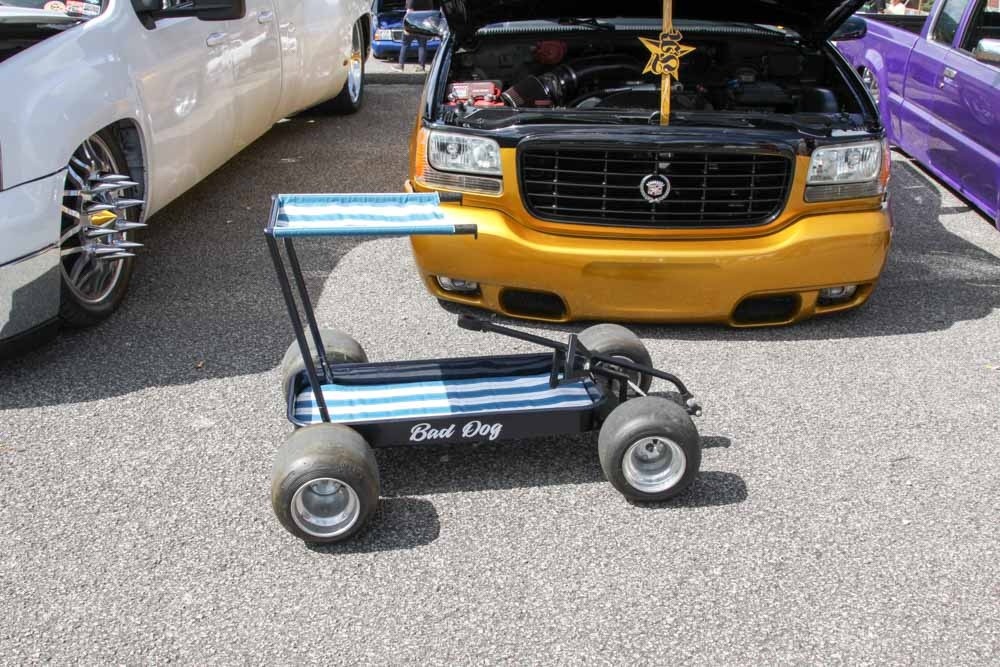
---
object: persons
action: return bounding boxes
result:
[392,0,435,73]
[860,0,925,15]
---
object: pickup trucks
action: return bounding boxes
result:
[829,0,1000,230]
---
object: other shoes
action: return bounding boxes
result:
[416,66,426,73]
[391,63,404,72]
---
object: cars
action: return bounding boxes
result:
[370,0,451,61]
[404,0,897,326]
[0,1,378,353]
